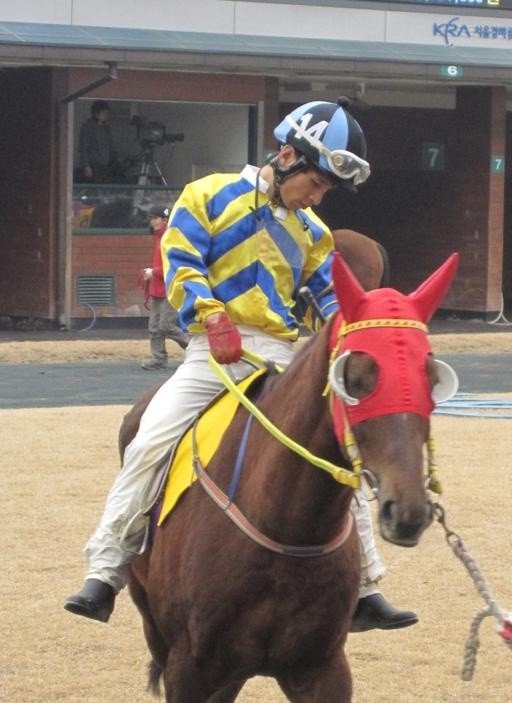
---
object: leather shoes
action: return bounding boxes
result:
[349,594,418,632]
[64,578,115,623]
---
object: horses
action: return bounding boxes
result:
[331,227,391,292]
[118,251,461,703]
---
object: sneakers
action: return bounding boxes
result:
[142,359,167,369]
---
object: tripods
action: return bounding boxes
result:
[131,146,176,216]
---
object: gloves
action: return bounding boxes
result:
[203,312,242,365]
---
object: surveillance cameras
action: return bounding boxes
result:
[353,80,366,100]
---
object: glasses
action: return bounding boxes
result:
[326,150,371,185]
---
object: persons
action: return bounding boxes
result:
[63,95,419,633]
[136,205,190,371]
[80,98,122,183]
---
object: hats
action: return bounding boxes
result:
[149,206,170,218]
[274,96,368,193]
